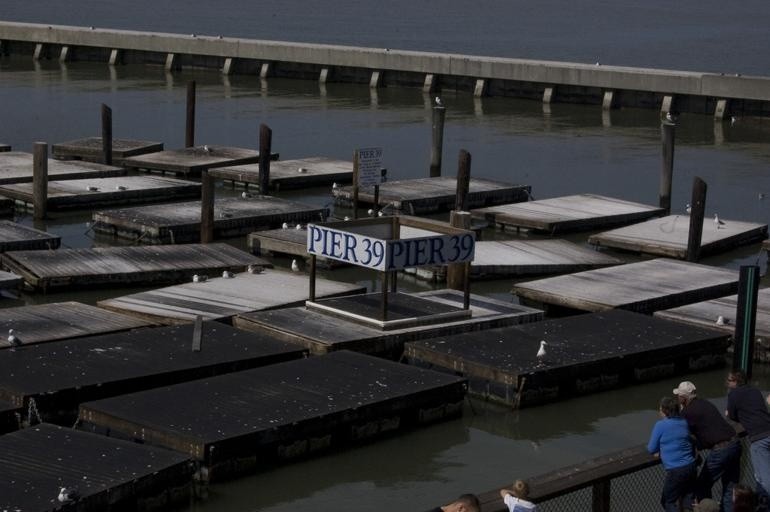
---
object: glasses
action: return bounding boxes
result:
[727,378,736,381]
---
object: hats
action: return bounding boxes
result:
[672,380,697,399]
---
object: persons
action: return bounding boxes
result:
[730,483,753,512]
[724,367,770,511]
[648,395,696,511]
[500,479,538,511]
[428,493,482,511]
[671,379,742,511]
[690,496,720,511]
